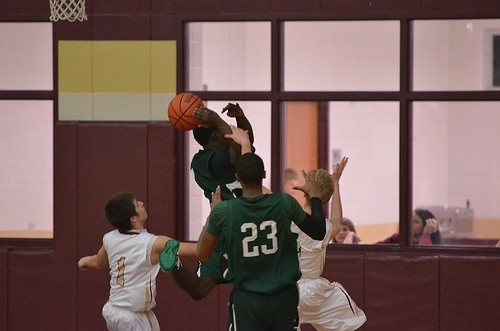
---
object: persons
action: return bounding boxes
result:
[377,209,442,245]
[159,102,367,331]
[78,191,198,331]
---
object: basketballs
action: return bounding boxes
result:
[167,92,204,132]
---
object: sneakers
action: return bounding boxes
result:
[159,239,183,272]
